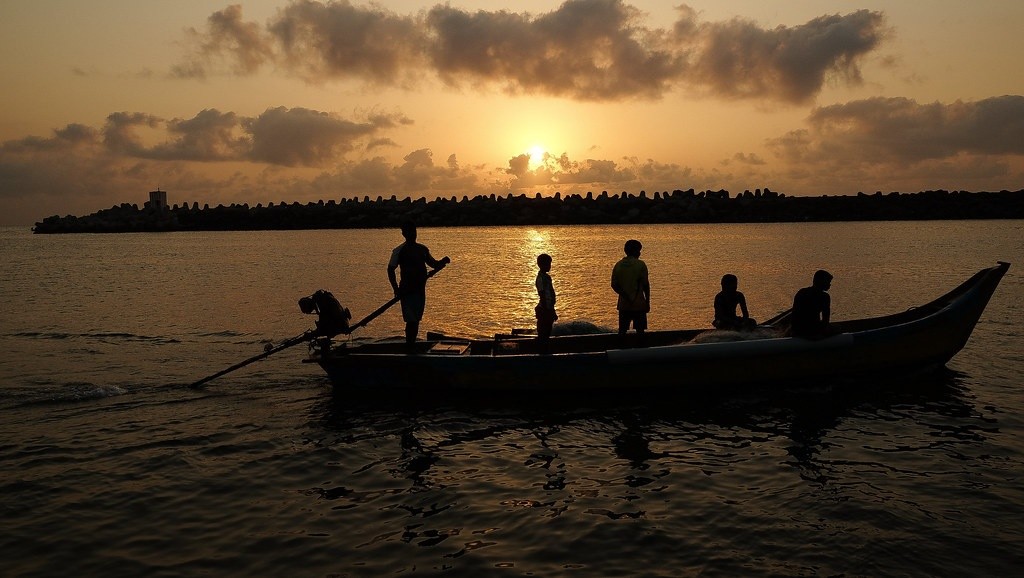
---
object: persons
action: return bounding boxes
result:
[387,224,446,354]
[535,254,558,353]
[714,274,757,332]
[611,240,650,349]
[791,269,840,340]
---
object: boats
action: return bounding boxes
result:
[299,259,1012,393]
